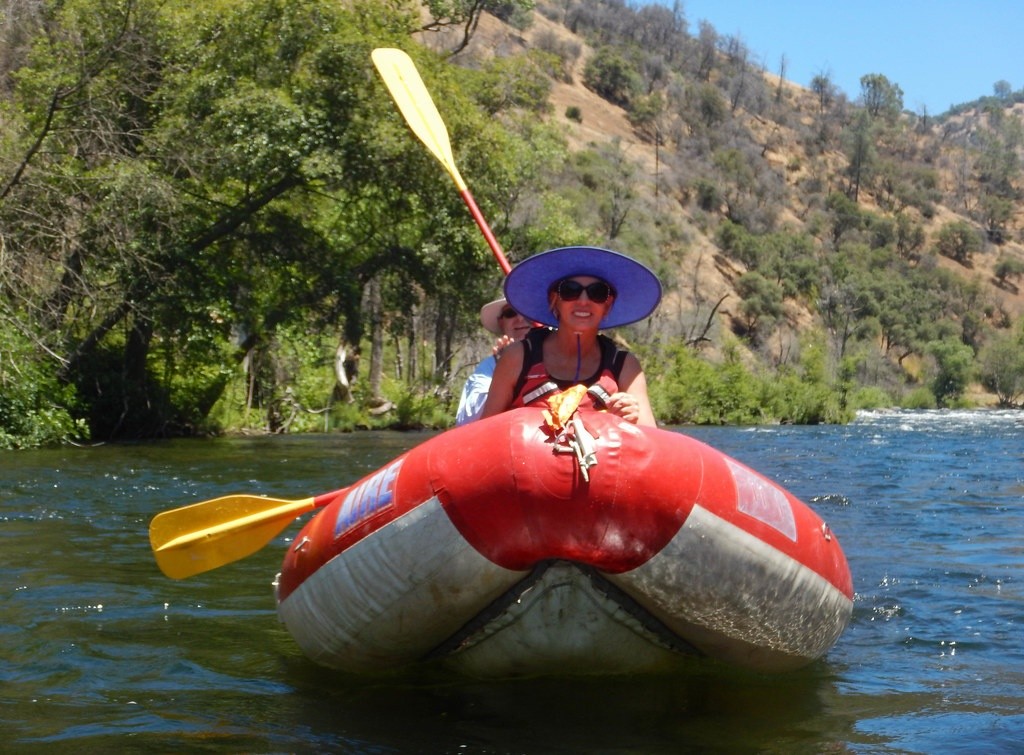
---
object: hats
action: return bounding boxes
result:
[480,298,509,338]
[504,246,662,330]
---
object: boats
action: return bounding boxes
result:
[271,407,852,682]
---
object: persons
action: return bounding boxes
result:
[455,298,560,425]
[481,246,662,430]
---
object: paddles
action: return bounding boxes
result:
[148,488,345,581]
[370,45,514,276]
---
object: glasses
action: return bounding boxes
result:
[548,279,617,305]
[498,308,518,319]
